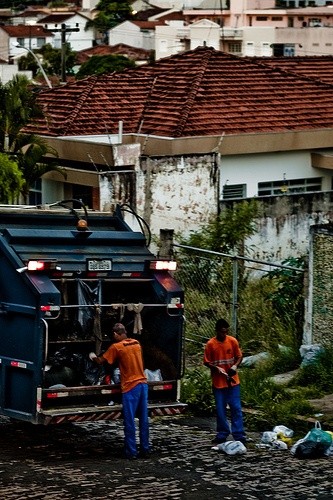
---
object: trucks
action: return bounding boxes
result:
[1,201,190,428]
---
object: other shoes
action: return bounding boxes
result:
[211,437,227,444]
[235,437,255,444]
[119,450,138,460]
[139,449,151,459]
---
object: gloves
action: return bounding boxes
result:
[216,364,238,378]
[88,351,97,361]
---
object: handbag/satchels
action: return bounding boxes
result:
[211,440,247,456]
[255,421,333,460]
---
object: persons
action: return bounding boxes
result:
[203,317,248,446]
[89,322,150,461]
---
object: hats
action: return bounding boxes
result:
[215,319,230,327]
[113,322,128,334]
